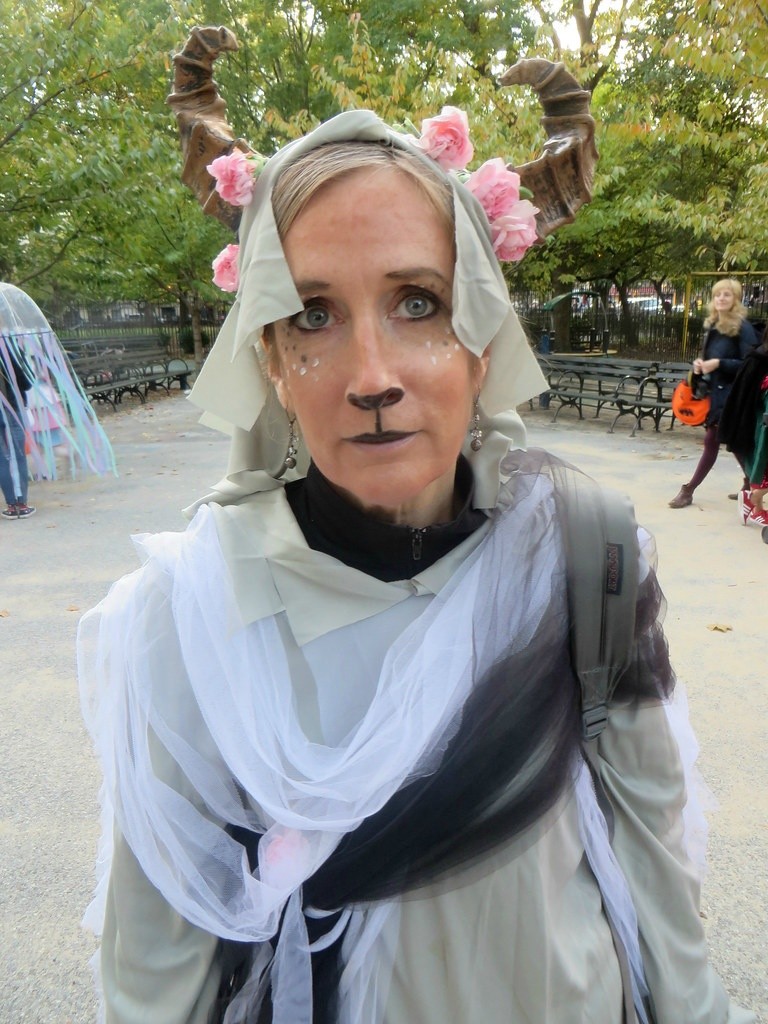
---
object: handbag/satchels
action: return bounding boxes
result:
[672,365,710,426]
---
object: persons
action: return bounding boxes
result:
[669,278,767,525]
[98,108,724,1024]
[0,280,49,521]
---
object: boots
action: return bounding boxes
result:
[728,478,750,500]
[668,484,692,507]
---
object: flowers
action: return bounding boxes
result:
[212,244,241,292]
[206,149,270,207]
[490,199,541,262]
[457,156,535,224]
[420,105,474,172]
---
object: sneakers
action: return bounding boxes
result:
[750,510,768,526]
[18,506,36,517]
[2,508,18,519]
[738,490,756,525]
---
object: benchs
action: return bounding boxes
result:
[61,333,195,413]
[536,355,655,434]
[538,359,679,431]
[630,362,694,437]
